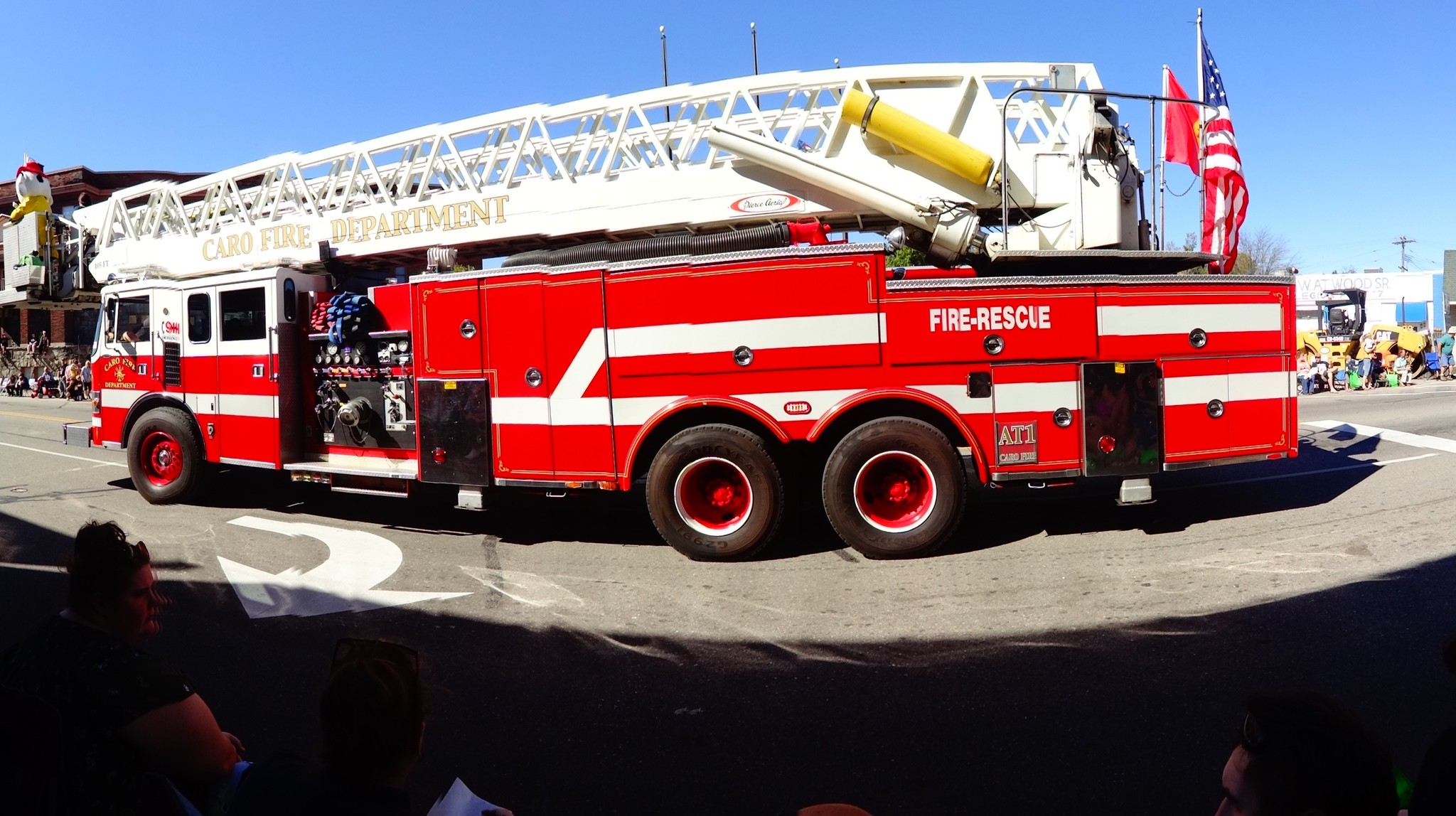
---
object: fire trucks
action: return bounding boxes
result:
[10,10,1301,560]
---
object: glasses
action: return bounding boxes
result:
[1315,356,1321,359]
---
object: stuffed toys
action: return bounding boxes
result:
[10,161,59,262]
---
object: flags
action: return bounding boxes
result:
[1200,28,1249,276]
[1163,69,1200,177]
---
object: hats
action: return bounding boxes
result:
[1448,326,1456,333]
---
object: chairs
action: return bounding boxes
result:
[1300,370,1346,392]
[0,378,35,397]
[1425,352,1441,380]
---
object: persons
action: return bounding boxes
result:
[120,318,150,342]
[1297,326,1456,395]
[0,330,92,401]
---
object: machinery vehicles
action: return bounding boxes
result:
[1296,286,1432,379]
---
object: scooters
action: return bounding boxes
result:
[30,376,60,399]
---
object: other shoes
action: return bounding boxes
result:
[1363,386,1368,391]
[1303,392,1308,395]
[1368,385,1374,390]
[1308,392,1312,394]
[44,353,46,356]
[1330,389,1338,393]
[1406,383,1411,387]
[1399,382,1405,387]
[1440,376,1447,381]
[33,353,34,355]
[27,353,30,355]
[1323,371,1327,377]
[1446,375,1453,380]
[0,393,23,397]
[3,353,5,356]
[66,394,91,401]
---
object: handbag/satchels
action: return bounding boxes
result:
[1386,366,1398,387]
[1348,367,1362,388]
